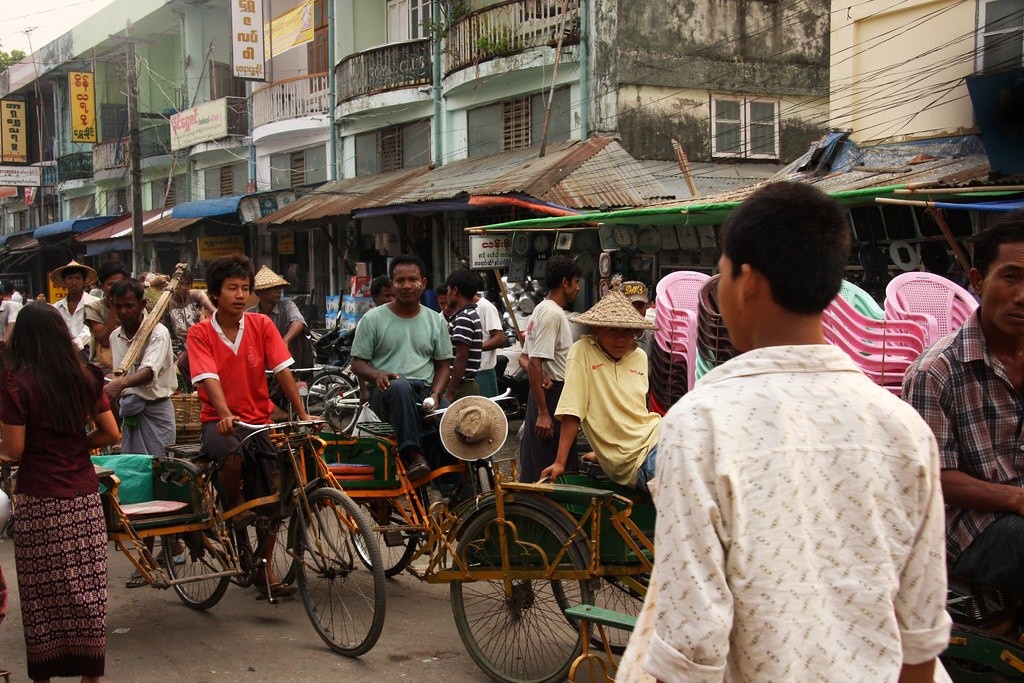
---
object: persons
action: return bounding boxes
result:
[246,265,316,405]
[901,223,1024,618]
[623,279,657,378]
[614,181,955,683]
[50,259,216,588]
[518,254,580,484]
[0,279,47,342]
[0,302,120,683]
[350,253,505,497]
[186,253,325,592]
[540,290,662,490]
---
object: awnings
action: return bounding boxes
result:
[0,132,1024,256]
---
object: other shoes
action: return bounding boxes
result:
[408,459,431,479]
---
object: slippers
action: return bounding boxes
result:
[254,582,297,600]
[233,510,256,533]
[155,556,186,568]
[125,571,149,588]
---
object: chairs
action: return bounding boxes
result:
[540,466,659,564]
[87,452,192,518]
[643,272,982,417]
[312,430,403,491]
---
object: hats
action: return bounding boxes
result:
[438,395,509,463]
[253,264,291,290]
[49,259,99,288]
[569,275,659,330]
[619,282,651,304]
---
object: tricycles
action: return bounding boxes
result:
[97,314,1024,683]
[91,417,387,658]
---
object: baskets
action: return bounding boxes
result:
[171,394,203,430]
